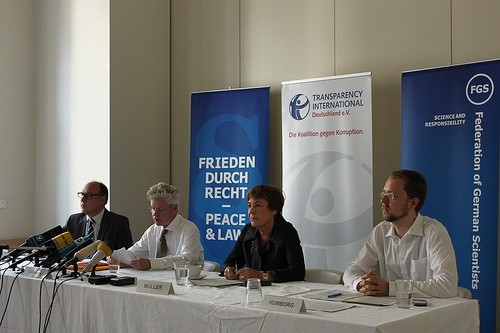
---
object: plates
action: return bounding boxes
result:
[188,275,207,280]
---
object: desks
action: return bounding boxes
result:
[0,254,478,333]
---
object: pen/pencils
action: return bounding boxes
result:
[328,293,342,298]
[235,260,238,272]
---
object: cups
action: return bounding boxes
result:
[108,255,120,276]
[395,280,414,310]
[246,277,263,308]
[189,265,202,277]
[173,261,190,287]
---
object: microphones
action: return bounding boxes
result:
[2,225,114,276]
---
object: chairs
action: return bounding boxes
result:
[203,261,221,272]
[303,269,343,284]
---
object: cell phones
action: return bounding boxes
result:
[413,300,427,307]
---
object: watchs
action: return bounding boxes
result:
[262,270,268,282]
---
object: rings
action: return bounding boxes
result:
[239,269,243,271]
[241,276,244,279]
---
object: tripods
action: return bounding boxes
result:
[64,262,90,281]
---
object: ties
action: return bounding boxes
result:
[87,219,95,240]
[159,229,169,257]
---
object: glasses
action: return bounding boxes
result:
[380,191,409,200]
[76,192,103,200]
[150,207,170,212]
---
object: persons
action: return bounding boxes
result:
[342,169,459,298]
[107,182,204,270]
[63,181,133,261]
[218,185,306,283]
